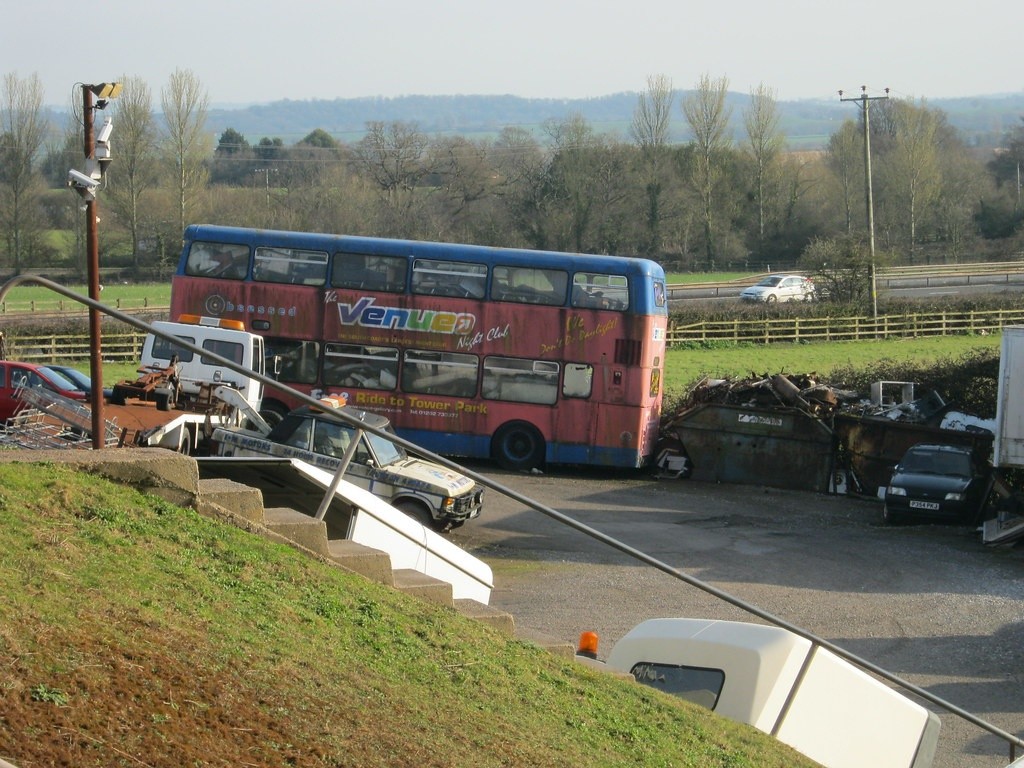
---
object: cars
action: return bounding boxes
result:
[0,361,115,425]
[885,446,979,523]
[739,275,816,304]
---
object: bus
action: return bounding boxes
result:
[170,222,667,472]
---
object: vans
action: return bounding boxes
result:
[137,313,265,413]
[575,617,942,768]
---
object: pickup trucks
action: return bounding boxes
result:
[211,384,485,532]
